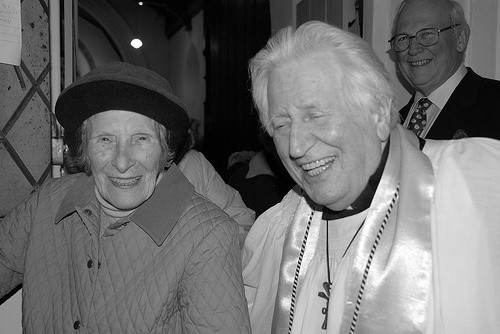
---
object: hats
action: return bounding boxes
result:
[55,61,192,164]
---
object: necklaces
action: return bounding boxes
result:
[318,210,366,330]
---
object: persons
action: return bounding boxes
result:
[0,62,254,334]
[226,0,500,334]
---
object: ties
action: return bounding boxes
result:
[406,97,433,137]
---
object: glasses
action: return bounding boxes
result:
[388,23,462,53]
